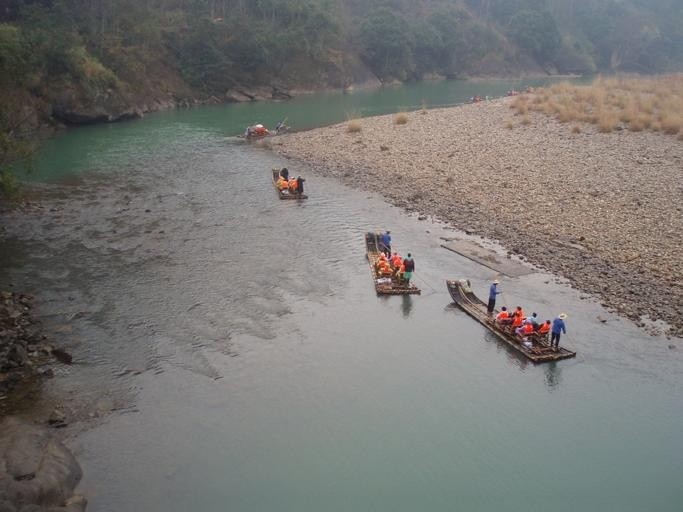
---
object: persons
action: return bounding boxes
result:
[276,167,305,195]
[496,306,567,348]
[377,231,415,290]
[487,280,500,313]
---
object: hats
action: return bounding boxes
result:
[558,313,568,320]
[492,279,500,284]
[385,228,392,232]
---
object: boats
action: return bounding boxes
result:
[224,113,289,143]
[272,167,309,201]
[446,278,577,364]
[363,229,421,296]
[465,82,532,104]
